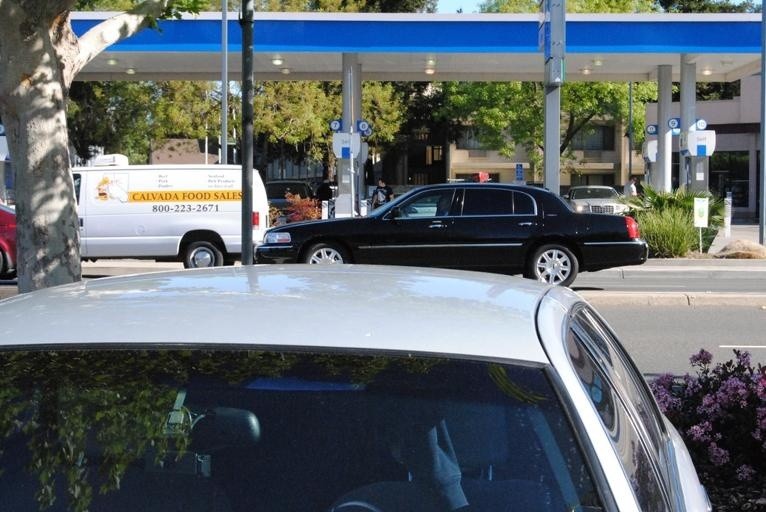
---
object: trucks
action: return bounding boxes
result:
[67,166,271,267]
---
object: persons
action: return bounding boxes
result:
[371,177,395,210]
[624,176,640,197]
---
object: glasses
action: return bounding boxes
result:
[633,179,637,182]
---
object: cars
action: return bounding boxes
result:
[0,263,714,512]
[254,182,648,286]
[563,186,631,216]
[265,178,336,224]
